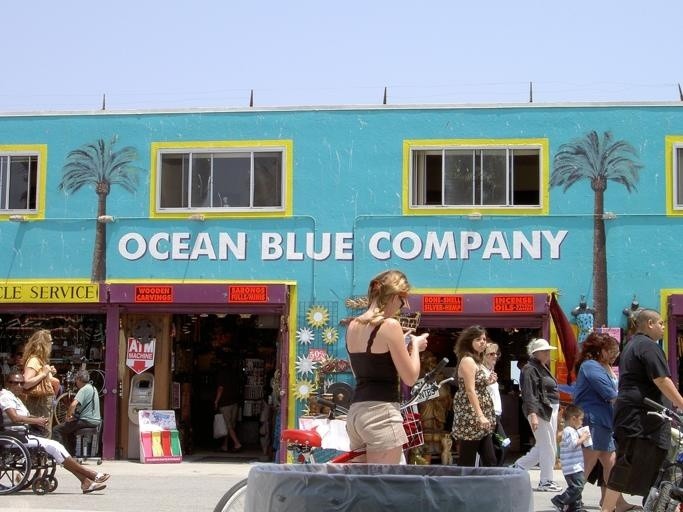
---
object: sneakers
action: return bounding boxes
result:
[538,479,563,492]
[551,499,568,511]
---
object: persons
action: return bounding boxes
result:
[571,299,597,344]
[344,268,431,466]
[588,335,621,507]
[479,341,508,467]
[550,403,592,512]
[212,355,244,454]
[599,307,683,512]
[449,321,499,467]
[567,331,644,512]
[506,337,564,492]
[622,300,646,343]
[0,325,112,494]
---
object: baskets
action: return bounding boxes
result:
[399,407,425,451]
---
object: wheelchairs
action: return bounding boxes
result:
[0,407,58,494]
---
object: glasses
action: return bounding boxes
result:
[6,380,25,386]
[399,296,406,308]
[485,352,501,357]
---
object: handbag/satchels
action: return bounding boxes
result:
[64,413,80,428]
[213,407,228,439]
[21,355,55,400]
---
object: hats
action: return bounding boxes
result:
[532,339,557,354]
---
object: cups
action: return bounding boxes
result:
[577,426,593,448]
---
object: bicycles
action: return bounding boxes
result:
[56,372,77,425]
[209,356,453,512]
[637,399,681,512]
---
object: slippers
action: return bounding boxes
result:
[95,472,111,483]
[82,481,107,494]
